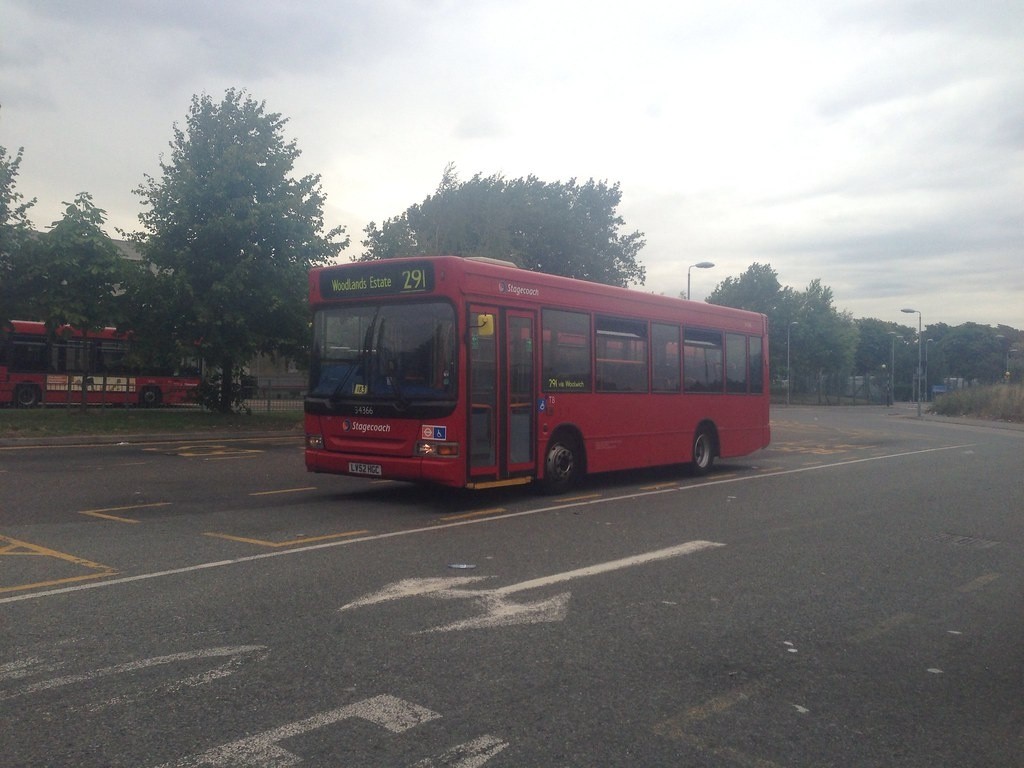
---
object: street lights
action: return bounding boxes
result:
[901,308,923,417]
[786,322,800,405]
[686,262,716,301]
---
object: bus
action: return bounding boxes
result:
[305,256,770,497]
[0,318,203,408]
[389,321,725,411]
